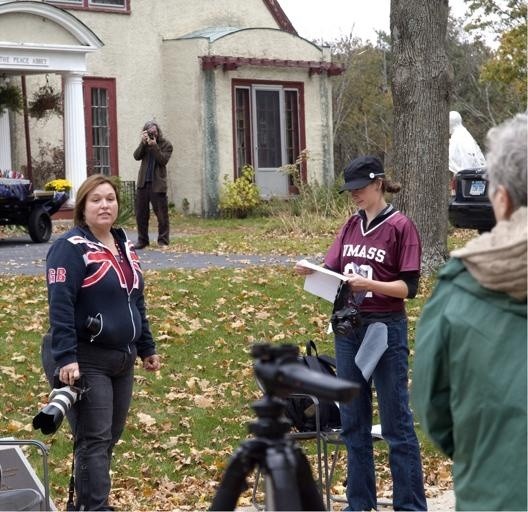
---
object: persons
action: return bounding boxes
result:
[131,120,173,249]
[446,112,486,197]
[411,108,527,511]
[294,156,432,512]
[41,171,162,511]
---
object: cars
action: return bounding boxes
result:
[448,167,497,234]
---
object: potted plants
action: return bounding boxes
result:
[1,81,63,121]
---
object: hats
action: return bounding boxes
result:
[338,157,386,194]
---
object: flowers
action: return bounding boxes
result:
[44,178,72,193]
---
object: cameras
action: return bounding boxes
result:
[330,306,361,335]
[148,131,157,140]
[32,367,88,435]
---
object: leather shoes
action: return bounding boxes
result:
[135,242,147,249]
[158,241,168,248]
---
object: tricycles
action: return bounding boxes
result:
[0,178,71,245]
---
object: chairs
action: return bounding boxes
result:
[250,355,394,512]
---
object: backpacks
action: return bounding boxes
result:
[289,339,341,432]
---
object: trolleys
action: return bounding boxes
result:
[0,436,59,511]
